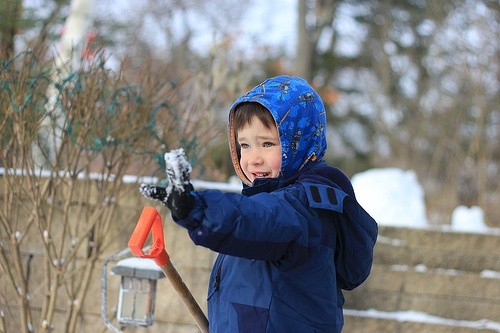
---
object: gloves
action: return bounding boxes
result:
[140,149,194,220]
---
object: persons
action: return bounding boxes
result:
[138,72,381,333]
